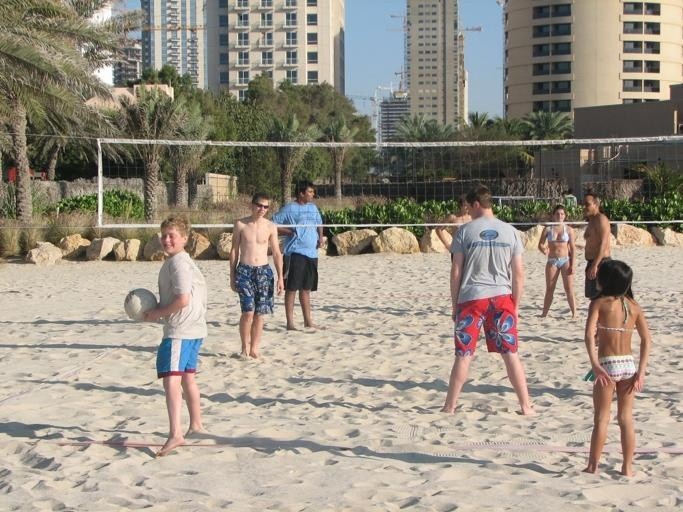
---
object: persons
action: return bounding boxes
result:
[270,179,325,334]
[435,195,474,262]
[581,259,652,477]
[230,192,284,360]
[440,185,536,415]
[537,204,576,318]
[581,191,613,346]
[142,212,205,458]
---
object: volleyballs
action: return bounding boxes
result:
[124,288,157,321]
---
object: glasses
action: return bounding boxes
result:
[254,202,269,209]
